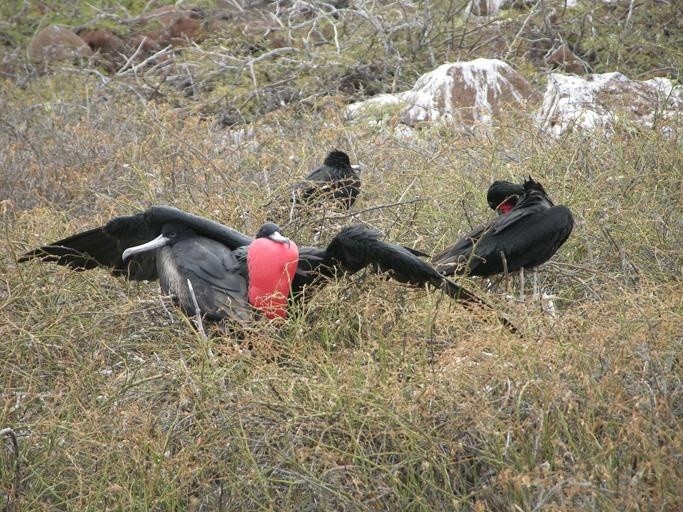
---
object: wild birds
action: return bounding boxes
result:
[16,203,525,357]
[238,148,362,233]
[427,174,576,280]
